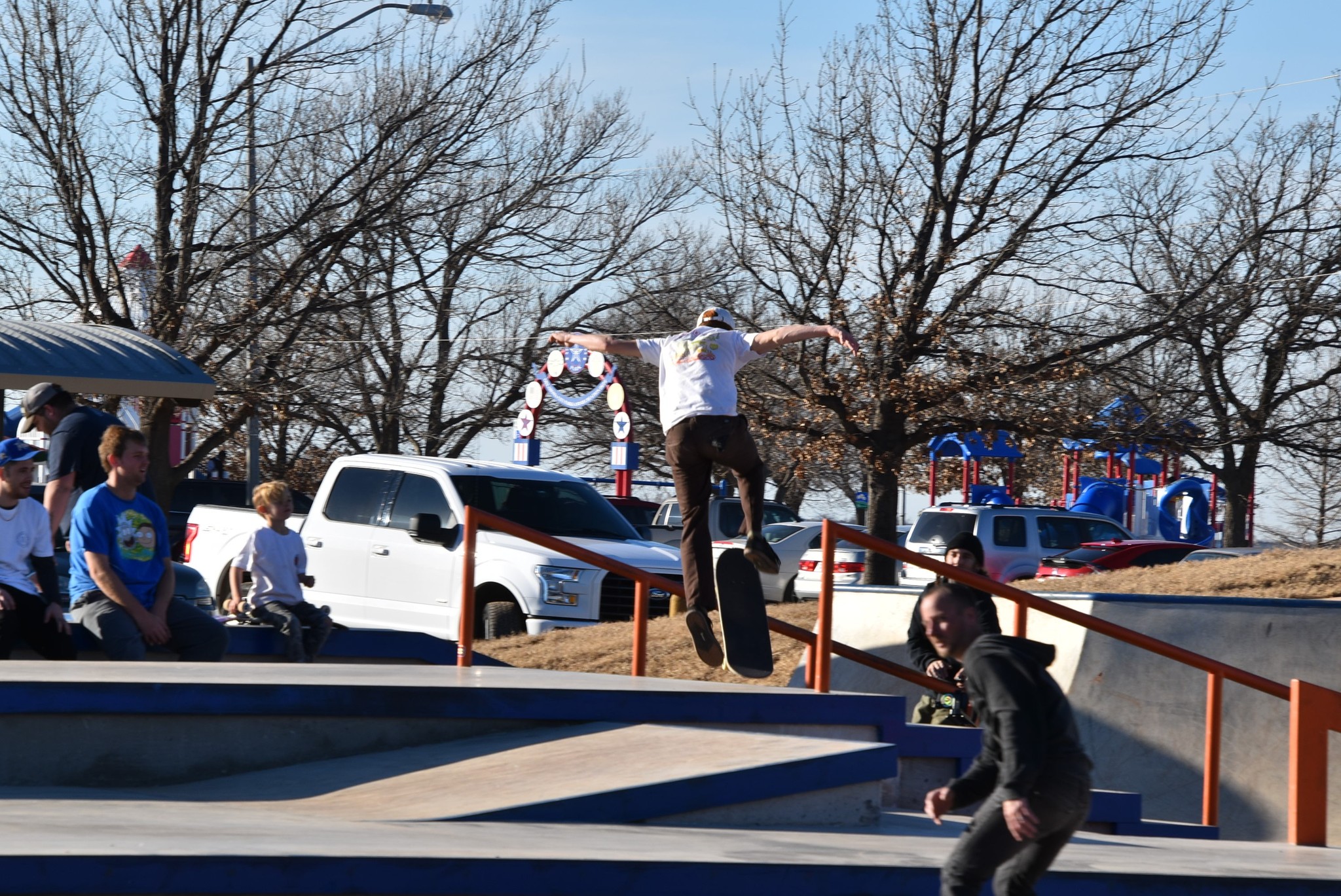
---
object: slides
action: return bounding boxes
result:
[1070,481,1124,519]
[982,490,1015,506]
[1158,479,1215,547]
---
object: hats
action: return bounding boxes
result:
[20,382,59,433]
[944,532,985,568]
[0,438,49,469]
[696,308,735,330]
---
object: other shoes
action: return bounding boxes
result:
[744,537,781,573]
[685,608,724,667]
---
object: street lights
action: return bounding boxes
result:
[243,3,456,510]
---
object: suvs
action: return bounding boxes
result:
[601,492,664,532]
[898,500,1139,589]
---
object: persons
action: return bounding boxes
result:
[228,481,333,662]
[0,439,79,660]
[67,426,231,663]
[547,307,859,666]
[20,382,156,560]
[908,531,1002,726]
[918,585,1091,896]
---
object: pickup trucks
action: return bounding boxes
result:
[182,454,686,646]
[629,492,803,549]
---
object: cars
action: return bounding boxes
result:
[1031,537,1210,580]
[1180,545,1269,563]
[167,479,316,561]
[794,522,915,603]
[706,521,868,604]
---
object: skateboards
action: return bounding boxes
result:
[716,547,774,679]
[222,598,349,630]
